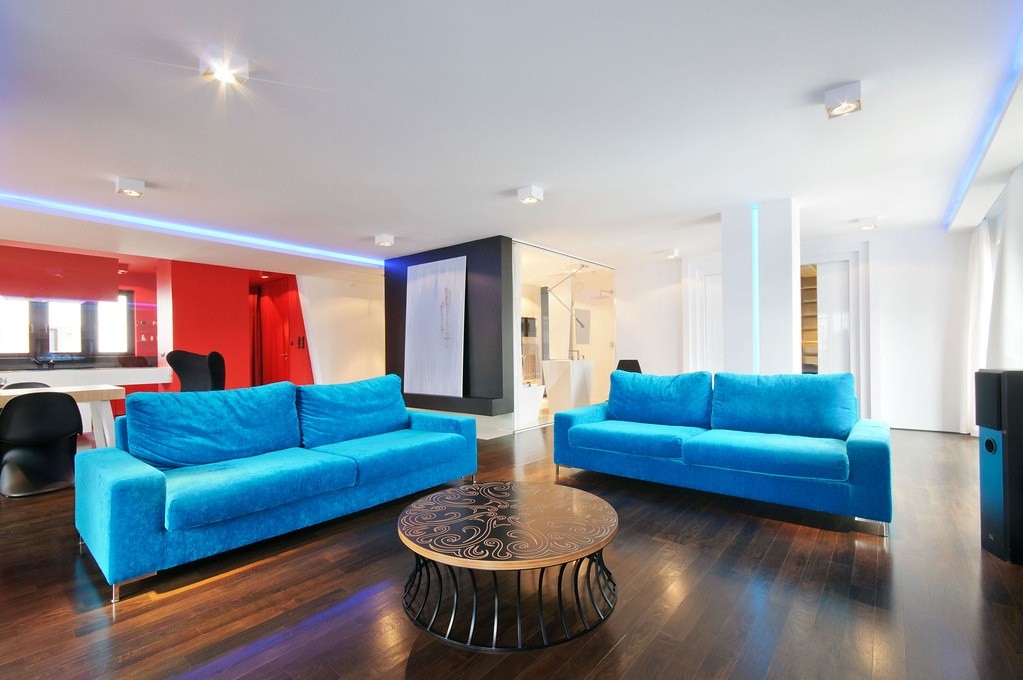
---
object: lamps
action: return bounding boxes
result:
[516,186,543,205]
[859,214,877,232]
[114,176,145,198]
[375,235,394,247]
[825,81,862,119]
[198,48,250,85]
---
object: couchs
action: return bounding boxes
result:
[74,373,477,603]
[554,370,893,532]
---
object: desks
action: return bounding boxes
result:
[541,359,589,415]
[0,384,125,448]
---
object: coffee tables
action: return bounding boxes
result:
[398,480,619,652]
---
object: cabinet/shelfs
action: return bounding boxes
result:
[801,260,818,374]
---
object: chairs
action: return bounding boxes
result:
[617,360,642,373]
[166,350,225,392]
[118,352,148,367]
[0,382,83,498]
